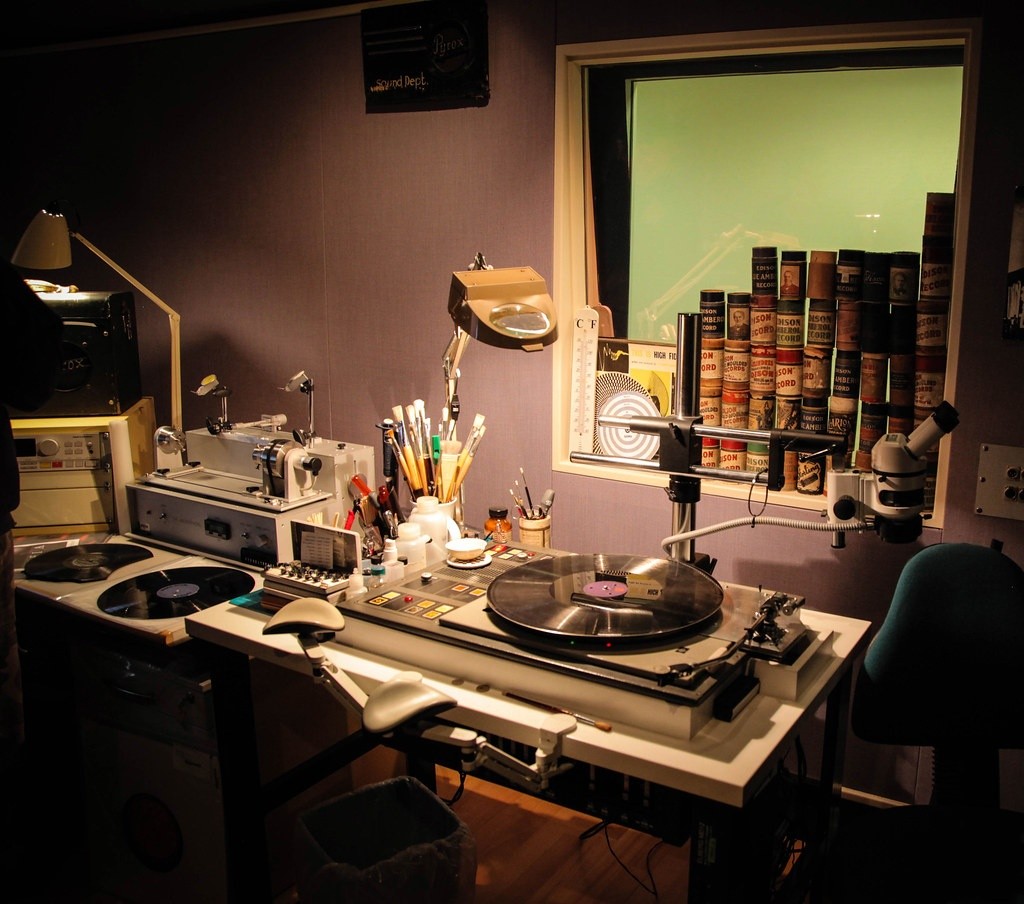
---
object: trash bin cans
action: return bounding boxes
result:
[298,774,476,904]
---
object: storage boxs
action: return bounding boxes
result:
[596,337,677,460]
[70,628,408,904]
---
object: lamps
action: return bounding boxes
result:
[10,209,183,437]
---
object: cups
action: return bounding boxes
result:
[409,495,457,521]
[518,514,551,549]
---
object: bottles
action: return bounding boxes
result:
[698,192,953,512]
[484,506,513,541]
[345,495,448,602]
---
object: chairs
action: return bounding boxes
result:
[808,543,1024,904]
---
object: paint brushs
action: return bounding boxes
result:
[404,397,437,497]
[510,481,529,520]
[446,413,486,502]
[387,404,425,502]
[433,406,455,497]
[503,692,613,732]
[520,468,536,516]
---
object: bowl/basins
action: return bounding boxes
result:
[445,538,487,560]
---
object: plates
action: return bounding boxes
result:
[447,554,492,569]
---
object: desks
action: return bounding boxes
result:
[184,542,874,904]
[0,530,263,904]
